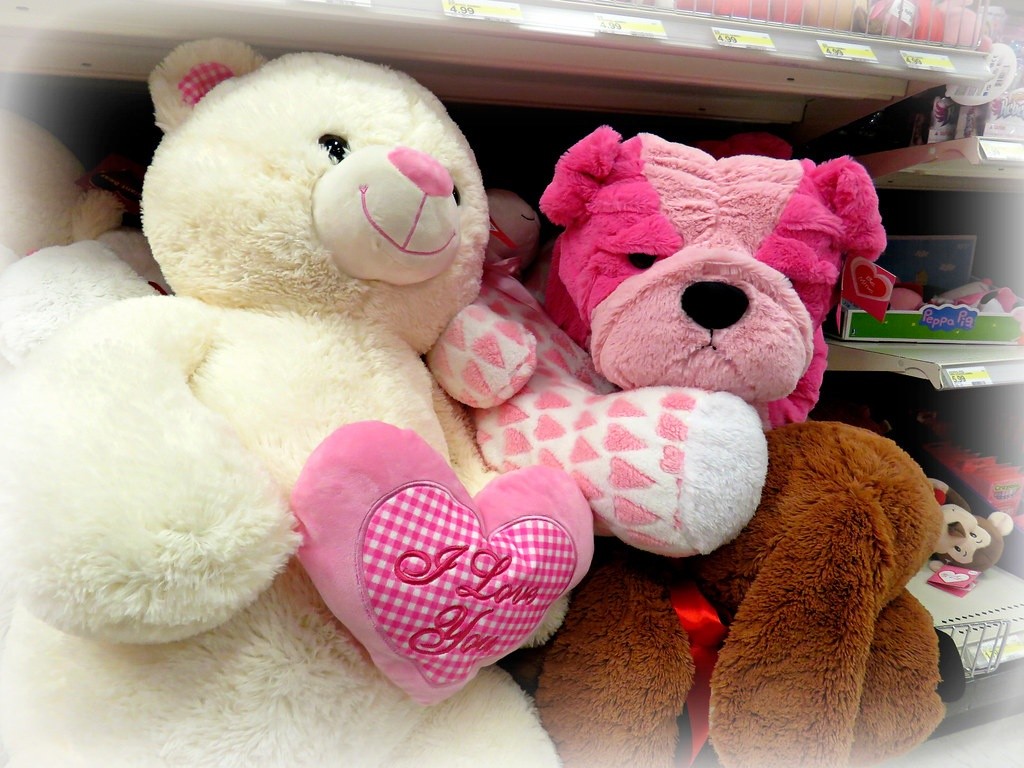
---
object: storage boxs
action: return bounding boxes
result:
[832,234,1021,347]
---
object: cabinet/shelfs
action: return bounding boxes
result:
[823,138,1024,741]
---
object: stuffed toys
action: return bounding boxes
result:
[539,123,886,429]
[928,477,1013,573]
[429,189,768,559]
[0,108,172,363]
[536,417,966,768]
[675,0,991,52]
[0,36,558,768]
